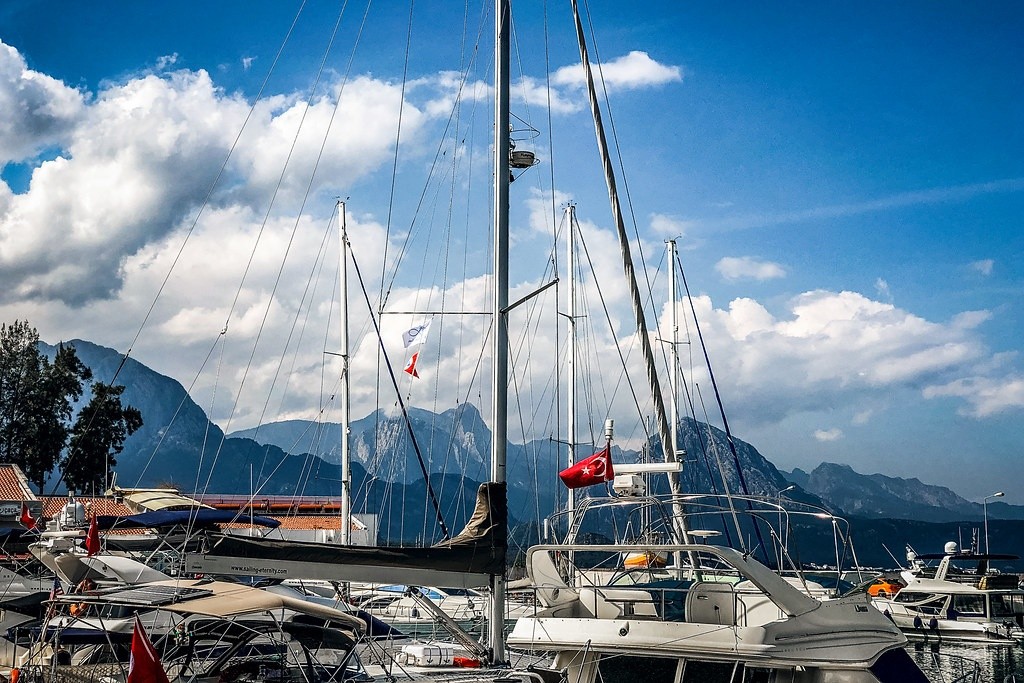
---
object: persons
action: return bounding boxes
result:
[50,643,73,666]
[883,610,898,628]
[173,624,190,661]
[914,613,938,632]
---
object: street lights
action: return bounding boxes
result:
[778,485,796,569]
[364,476,380,514]
[984,493,1005,573]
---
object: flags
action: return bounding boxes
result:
[20,499,36,530]
[402,316,434,349]
[46,577,64,619]
[128,611,170,683]
[559,444,615,489]
[85,510,101,558]
[404,351,420,379]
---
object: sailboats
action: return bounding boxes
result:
[0,0,1024,682]
